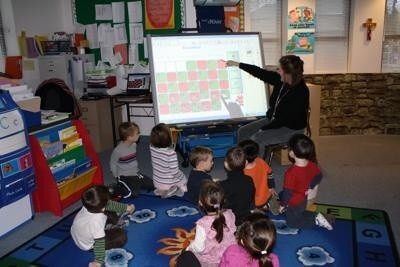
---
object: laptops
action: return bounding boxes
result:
[114,73,151,97]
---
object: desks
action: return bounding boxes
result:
[110,90,158,150]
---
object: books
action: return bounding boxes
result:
[1,76,146,189]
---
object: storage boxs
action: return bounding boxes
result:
[77,98,124,151]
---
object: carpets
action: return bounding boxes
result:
[0,180,399,266]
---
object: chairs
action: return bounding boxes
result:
[262,106,313,168]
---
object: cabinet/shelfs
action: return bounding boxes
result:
[31,119,105,217]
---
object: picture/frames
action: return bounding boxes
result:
[127,72,151,91]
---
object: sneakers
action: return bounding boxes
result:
[153,184,177,199]
[106,183,122,196]
[112,219,129,229]
[315,211,336,232]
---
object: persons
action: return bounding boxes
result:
[187,146,215,205]
[278,133,337,232]
[173,178,239,267]
[217,211,280,267]
[237,139,278,210]
[215,147,255,232]
[219,54,309,160]
[69,183,135,267]
[147,122,188,198]
[105,121,154,200]
